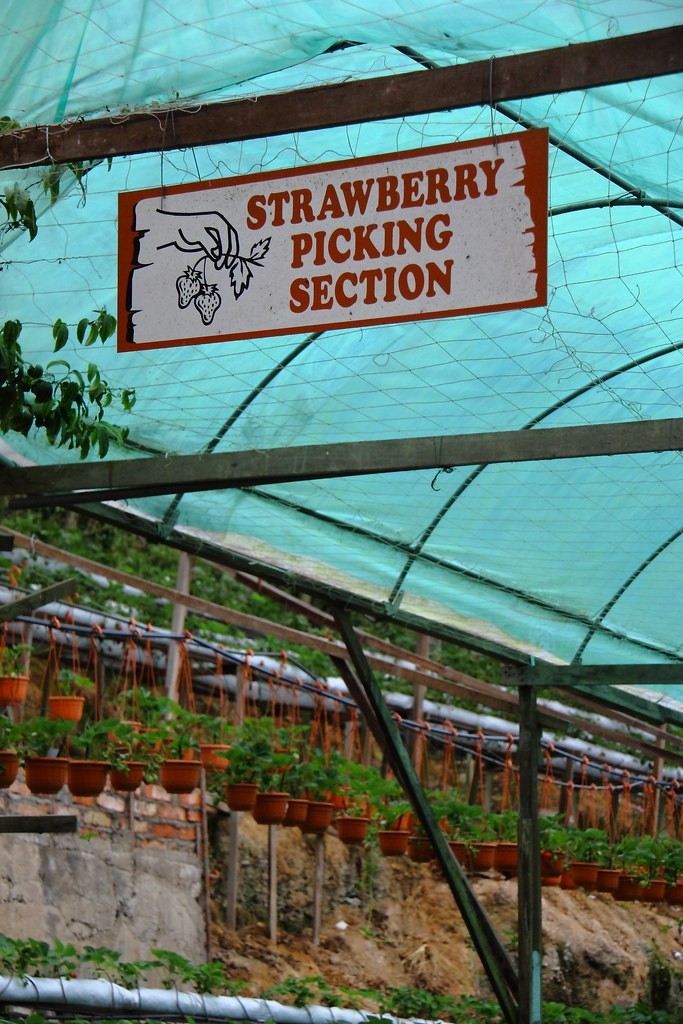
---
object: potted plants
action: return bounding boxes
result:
[0,643,683,905]
